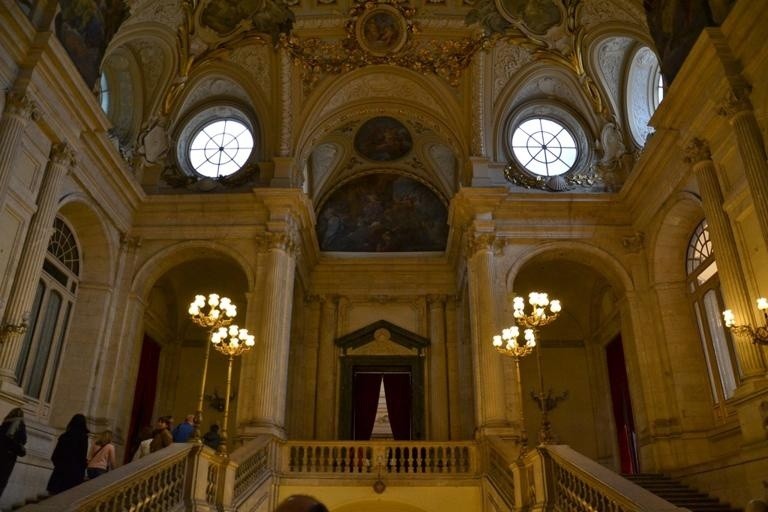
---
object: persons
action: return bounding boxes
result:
[131,426,157,462]
[381,25,391,47]
[149,415,173,452]
[86,429,117,481]
[45,412,90,497]
[366,19,379,43]
[1,407,27,498]
[171,413,197,442]
[202,423,220,450]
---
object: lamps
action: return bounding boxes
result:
[719,297,768,345]
[0,309,31,345]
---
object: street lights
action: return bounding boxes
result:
[189,292,236,454]
[489,326,537,459]
[209,321,256,454]
[510,290,561,459]
